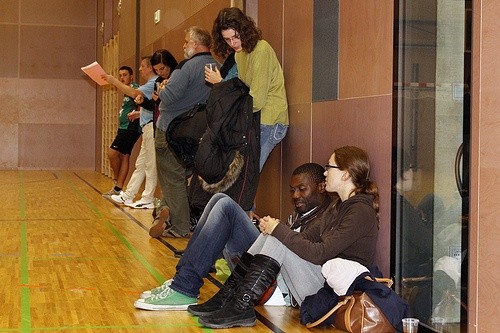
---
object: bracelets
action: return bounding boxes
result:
[219,78,223,82]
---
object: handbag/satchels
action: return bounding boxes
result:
[165,102,207,169]
[305,291,393,333]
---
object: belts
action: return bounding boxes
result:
[141,120,153,128]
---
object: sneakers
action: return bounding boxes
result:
[125,200,155,209]
[142,278,174,299]
[133,286,197,311]
[102,187,121,197]
[110,192,133,206]
[149,206,170,238]
[161,226,190,237]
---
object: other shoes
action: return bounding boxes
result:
[174,249,186,258]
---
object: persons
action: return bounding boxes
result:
[390,152,463,304]
[99,7,288,281]
[133,161,332,311]
[188,145,379,328]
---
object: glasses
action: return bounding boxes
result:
[324,164,344,171]
[223,33,240,43]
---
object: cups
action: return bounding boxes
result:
[206,62,217,71]
[402,318,419,333]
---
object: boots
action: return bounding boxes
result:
[188,250,255,316]
[198,254,282,329]
